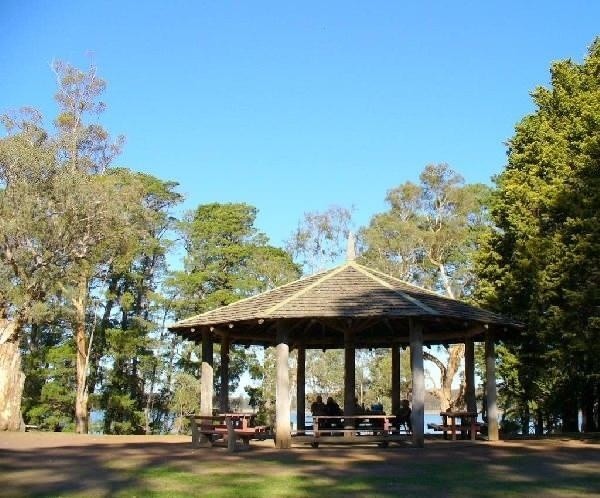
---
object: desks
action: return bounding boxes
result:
[440,411,478,440]
[185,412,256,447]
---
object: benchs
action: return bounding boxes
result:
[427,422,488,441]
[310,414,396,449]
[197,424,270,446]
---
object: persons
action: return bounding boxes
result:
[311,395,411,436]
